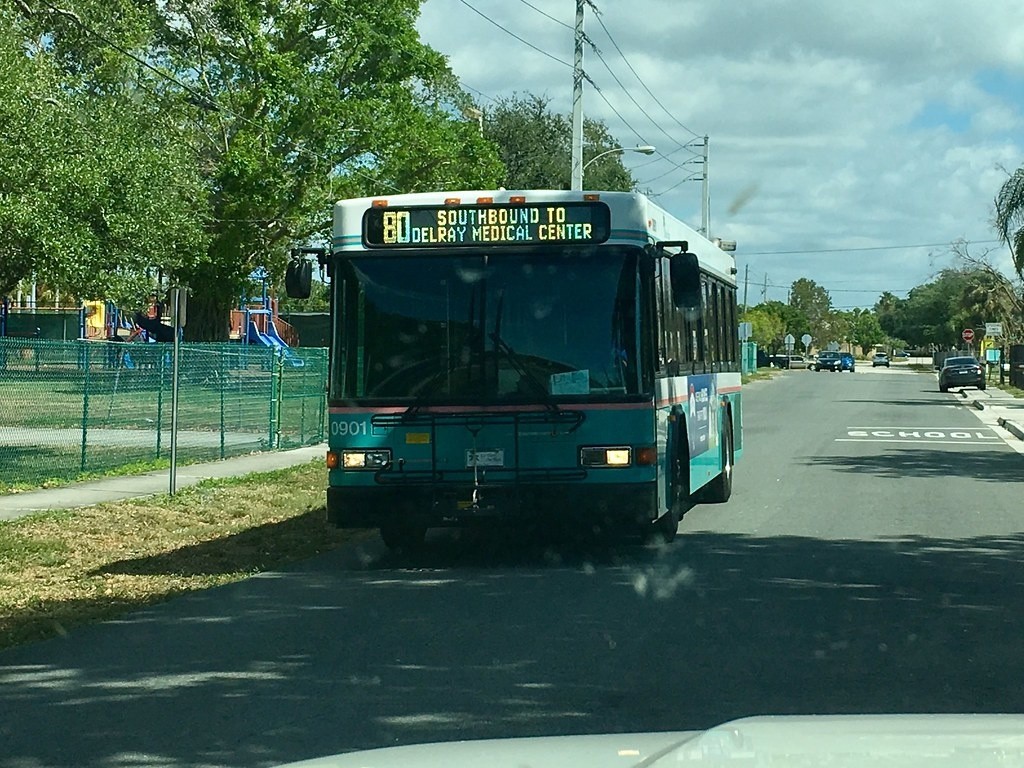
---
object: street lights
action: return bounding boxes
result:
[570,144,658,191]
[463,104,484,143]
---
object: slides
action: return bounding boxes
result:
[119,345,135,370]
[249,321,305,367]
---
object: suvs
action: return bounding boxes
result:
[872,352,890,368]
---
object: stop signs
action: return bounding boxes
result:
[962,328,975,341]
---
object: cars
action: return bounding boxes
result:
[814,351,842,373]
[895,349,911,358]
[838,351,856,372]
[789,355,817,371]
[934,355,987,392]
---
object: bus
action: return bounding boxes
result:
[284,187,746,553]
